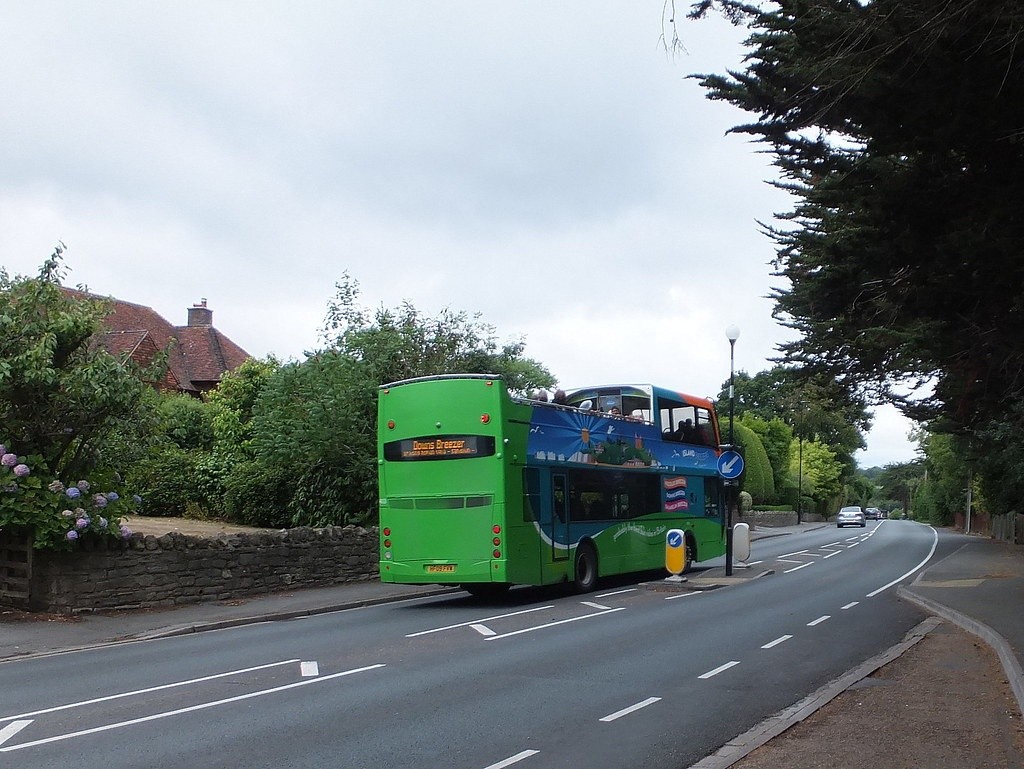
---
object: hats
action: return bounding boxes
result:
[578,400,592,413]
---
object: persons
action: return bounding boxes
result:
[532,390,622,421]
[675,418,710,444]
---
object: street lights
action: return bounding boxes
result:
[726,323,741,575]
[798,401,810,524]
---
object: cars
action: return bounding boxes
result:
[866,508,880,521]
[837,505,866,528]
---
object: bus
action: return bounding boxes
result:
[377,372,723,599]
